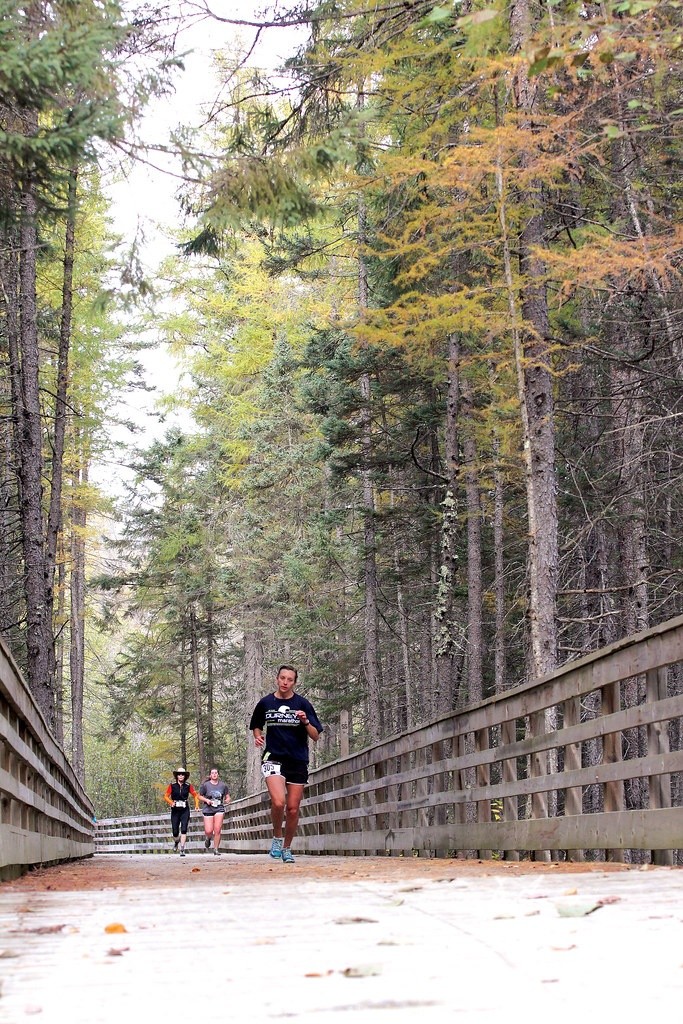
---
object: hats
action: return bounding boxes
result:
[173,768,190,781]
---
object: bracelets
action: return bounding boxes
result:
[304,719,309,726]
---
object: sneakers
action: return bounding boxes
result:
[180,850,185,856]
[214,849,221,855]
[281,846,295,863]
[205,837,211,848]
[173,841,179,852]
[270,836,283,860]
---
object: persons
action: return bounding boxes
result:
[249,665,323,863]
[197,767,231,855]
[164,768,198,856]
